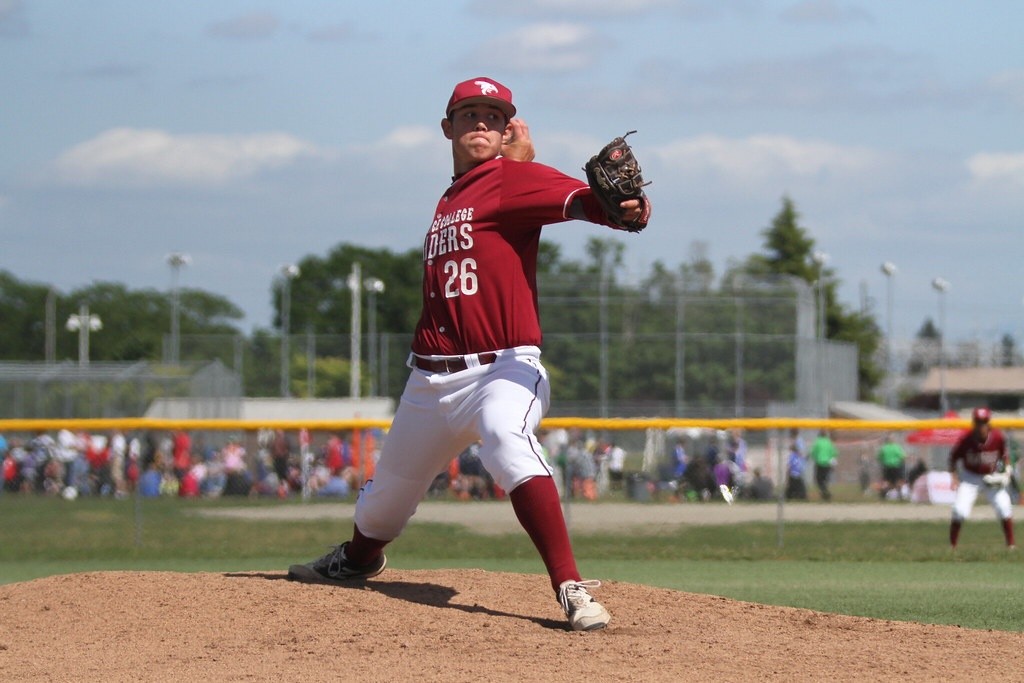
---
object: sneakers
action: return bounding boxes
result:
[556,579,612,633]
[288,540,386,580]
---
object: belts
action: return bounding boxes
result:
[412,353,497,374]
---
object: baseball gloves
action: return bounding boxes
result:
[584,136,650,231]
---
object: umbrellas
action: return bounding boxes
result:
[908,410,971,446]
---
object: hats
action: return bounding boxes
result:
[972,407,991,423]
[445,77,516,120]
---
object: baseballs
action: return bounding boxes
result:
[504,132,514,144]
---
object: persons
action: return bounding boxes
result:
[668,428,775,501]
[877,433,909,500]
[0,427,374,500]
[287,77,652,631]
[542,428,626,499]
[784,428,807,501]
[948,407,1015,547]
[805,429,837,500]
[457,440,489,499]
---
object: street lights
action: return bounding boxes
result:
[67,307,104,375]
[929,277,952,407]
[363,276,384,401]
[165,251,194,375]
[878,261,903,407]
[277,262,304,400]
